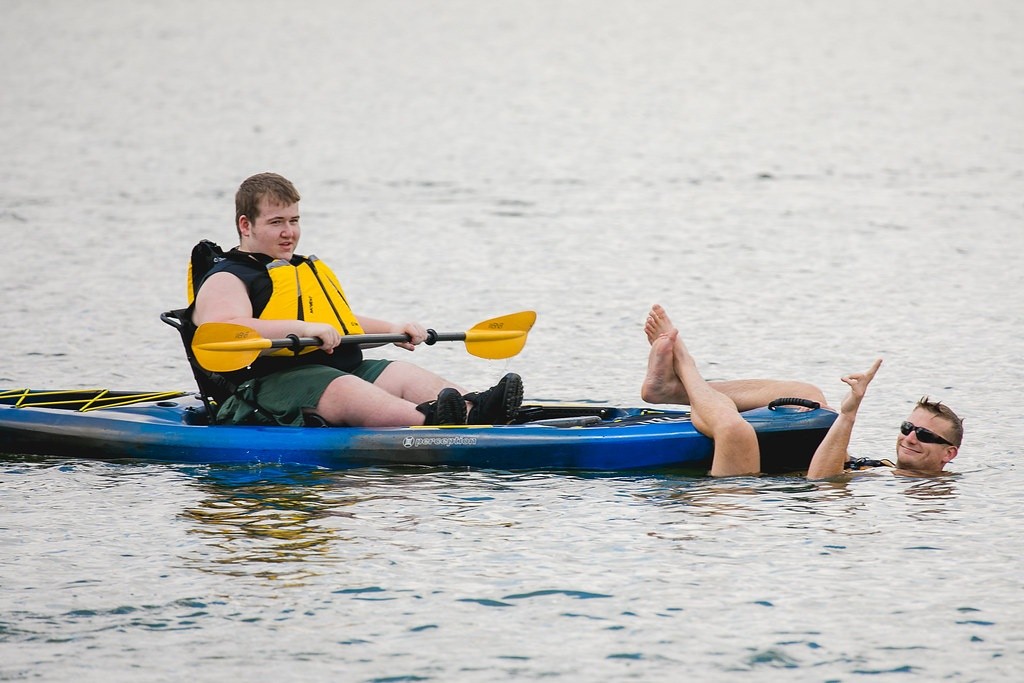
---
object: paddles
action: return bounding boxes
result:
[191,311,537,372]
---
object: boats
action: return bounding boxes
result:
[0,387,840,477]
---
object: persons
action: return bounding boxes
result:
[641,303,963,478]
[160,172,525,427]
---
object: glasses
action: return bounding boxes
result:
[901,421,955,446]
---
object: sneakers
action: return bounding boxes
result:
[416,387,467,426]
[462,373,525,425]
